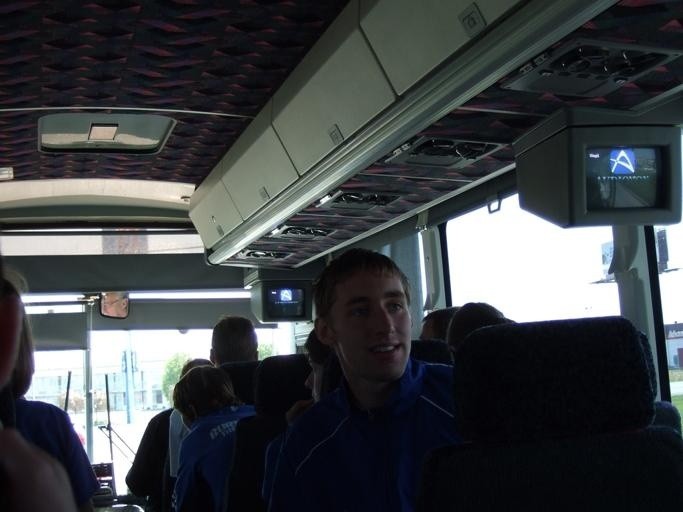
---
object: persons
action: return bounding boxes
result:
[0,292,77,512]
[174,362,261,510]
[419,305,459,340]
[124,359,210,511]
[272,251,485,508]
[100,293,130,318]
[263,324,333,493]
[443,304,506,363]
[206,318,261,367]
[0,251,78,511]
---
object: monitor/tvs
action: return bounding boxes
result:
[515,120,681,228]
[251,281,316,324]
